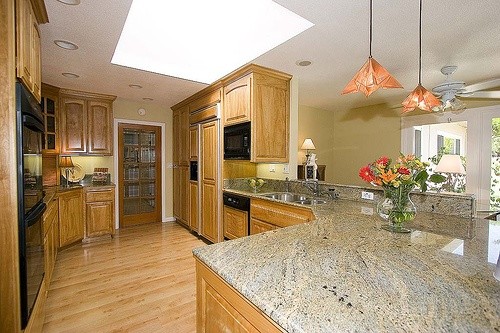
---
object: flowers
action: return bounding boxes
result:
[356,149,431,225]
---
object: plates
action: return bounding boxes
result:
[62,162,86,182]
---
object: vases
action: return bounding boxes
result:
[378,185,417,224]
[375,194,394,221]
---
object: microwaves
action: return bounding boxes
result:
[223,121,251,161]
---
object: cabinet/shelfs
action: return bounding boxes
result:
[172,64,310,248]
[297,164,327,182]
[0,0,116,333]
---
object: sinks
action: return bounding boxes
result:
[260,193,308,202]
[293,197,324,206]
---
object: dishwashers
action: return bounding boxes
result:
[223,193,250,241]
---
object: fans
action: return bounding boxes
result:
[387,65,500,110]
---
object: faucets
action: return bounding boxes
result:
[302,181,319,197]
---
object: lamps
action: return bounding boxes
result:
[434,153,467,193]
[429,92,467,117]
[402,0,441,114]
[300,138,316,164]
[58,155,75,189]
[338,0,404,97]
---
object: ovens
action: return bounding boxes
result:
[15,81,48,330]
